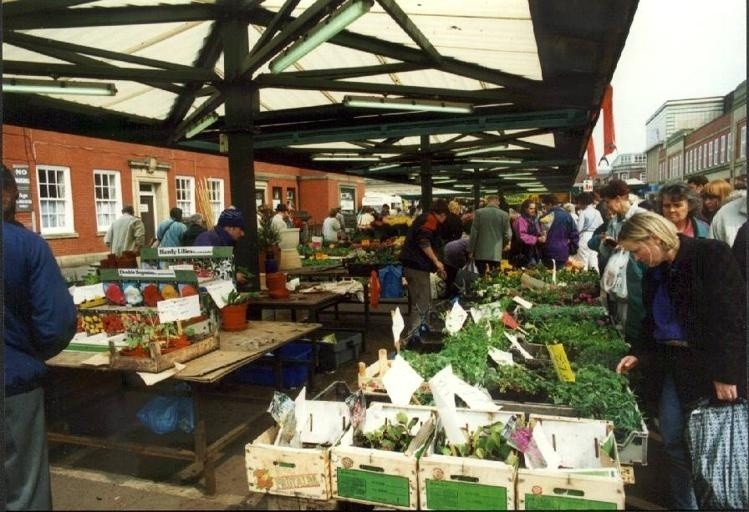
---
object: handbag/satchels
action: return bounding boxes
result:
[456,260,480,289]
[681,396,749,512]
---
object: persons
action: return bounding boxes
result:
[155,208,191,251]
[2,164,80,512]
[266,200,390,251]
[615,213,747,512]
[102,204,149,256]
[573,179,748,348]
[189,206,248,247]
[399,190,577,345]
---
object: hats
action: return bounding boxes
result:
[277,203,287,211]
[219,205,245,227]
